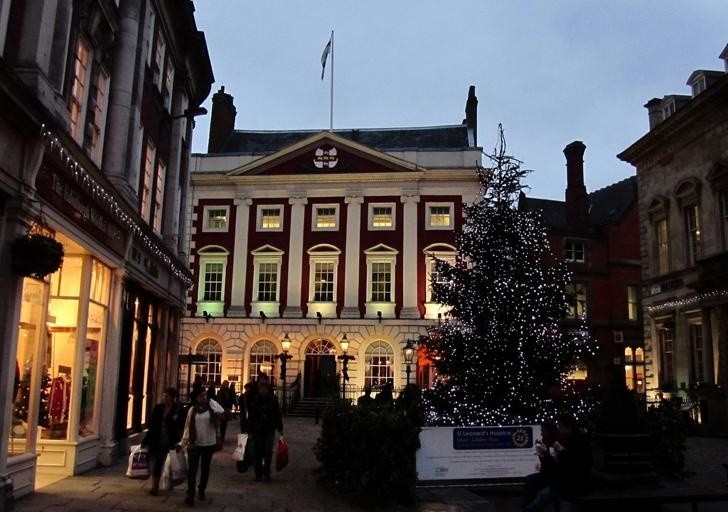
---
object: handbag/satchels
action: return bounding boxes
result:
[158,450,189,491]
[216,436,224,451]
[275,440,289,472]
[231,433,249,473]
[125,444,151,480]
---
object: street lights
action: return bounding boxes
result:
[279,333,293,416]
[403,337,413,383]
[337,333,356,398]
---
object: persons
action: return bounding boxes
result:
[358,384,405,405]
[141,381,283,506]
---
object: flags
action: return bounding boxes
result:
[320,35,331,79]
